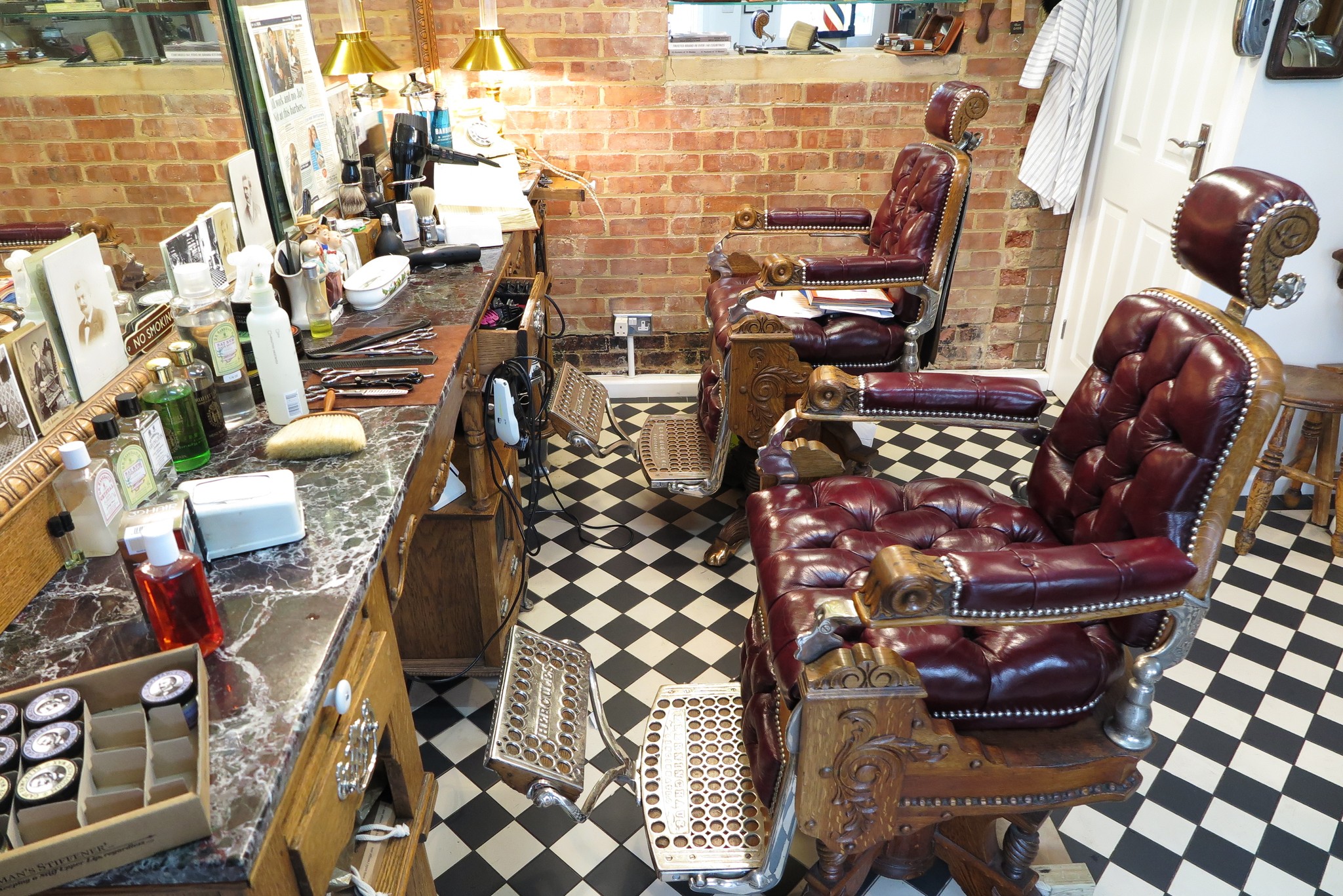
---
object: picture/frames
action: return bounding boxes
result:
[1265,1,1342,81]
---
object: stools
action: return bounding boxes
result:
[1234,361,1342,563]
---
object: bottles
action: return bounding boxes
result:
[50,234,334,564]
[132,523,222,668]
[373,213,406,259]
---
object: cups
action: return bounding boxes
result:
[407,84,453,150]
[415,213,438,247]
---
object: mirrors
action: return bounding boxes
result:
[2,0,257,535]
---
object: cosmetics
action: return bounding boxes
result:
[142,357,210,472]
[87,413,160,512]
[53,441,126,557]
[134,524,223,658]
[414,90,453,150]
[1,669,195,814]
[327,217,362,276]
[301,261,333,338]
[170,261,256,431]
[49,516,77,571]
[166,340,228,449]
[375,213,405,258]
[375,198,403,241]
[230,288,304,404]
[359,167,383,220]
[113,392,178,498]
[361,153,386,202]
[58,511,85,564]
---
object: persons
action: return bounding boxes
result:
[308,128,320,171]
[220,220,236,259]
[311,125,325,169]
[267,27,289,92]
[289,144,302,211]
[30,342,58,421]
[74,281,108,346]
[242,175,261,224]
[335,111,348,160]
[343,102,356,160]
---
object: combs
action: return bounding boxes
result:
[277,249,289,276]
[298,190,312,271]
[306,318,431,359]
[285,233,294,275]
[297,356,438,370]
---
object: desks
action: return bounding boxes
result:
[3,154,560,896]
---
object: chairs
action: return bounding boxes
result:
[541,80,990,557]
[489,166,1326,892]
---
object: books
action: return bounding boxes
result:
[162,42,223,65]
[792,288,893,320]
[667,30,731,56]
[433,163,540,235]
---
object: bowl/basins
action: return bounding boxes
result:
[344,252,410,313]
